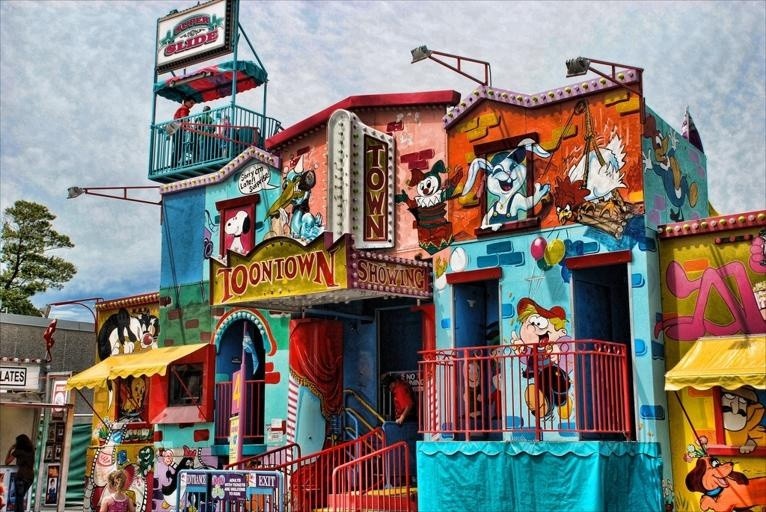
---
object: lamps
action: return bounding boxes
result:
[411,45,492,86]
[565,56,646,124]
[40,297,103,333]
[66,185,163,225]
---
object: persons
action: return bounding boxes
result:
[213,115,239,159]
[47,478,56,493]
[194,105,216,162]
[98,469,137,511]
[4,433,35,511]
[381,373,417,426]
[170,96,197,168]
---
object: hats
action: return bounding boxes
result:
[383,375,400,392]
